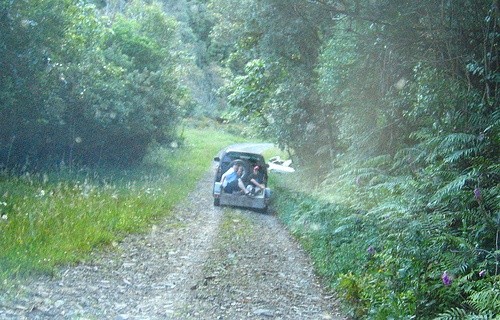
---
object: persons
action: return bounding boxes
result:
[246,165,266,200]
[220,161,252,198]
[236,166,246,181]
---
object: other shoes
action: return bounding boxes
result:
[233,191,241,196]
[245,191,256,199]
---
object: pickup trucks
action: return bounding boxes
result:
[213,150,270,212]
